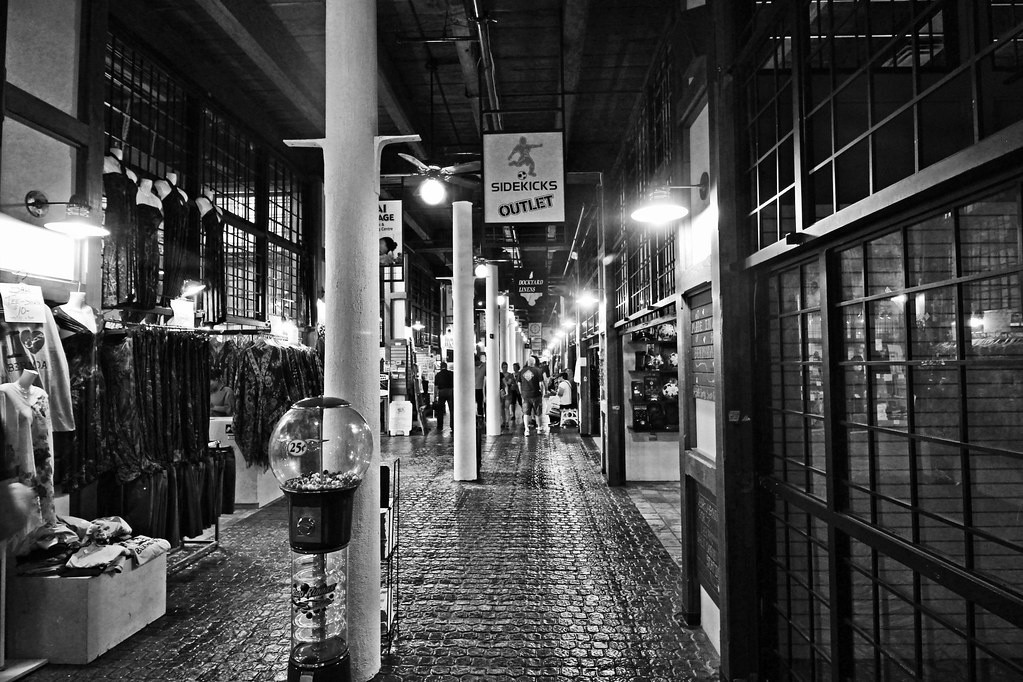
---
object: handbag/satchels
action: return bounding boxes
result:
[546,392,560,417]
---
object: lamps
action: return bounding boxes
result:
[420,177,447,205]
[153,280,206,299]
[24,191,112,238]
[631,172,710,224]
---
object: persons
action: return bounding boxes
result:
[103,148,227,324]
[434,356,578,437]
[0,369,57,527]
[51,292,112,495]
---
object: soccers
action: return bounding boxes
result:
[645,323,679,399]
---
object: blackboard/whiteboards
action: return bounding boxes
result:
[689,291,715,402]
[693,479,720,609]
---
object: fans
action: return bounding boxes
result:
[381,151,482,188]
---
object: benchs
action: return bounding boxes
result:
[557,407,579,428]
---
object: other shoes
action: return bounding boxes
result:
[436,427,443,430]
[524,430,530,436]
[548,421,559,426]
[537,430,541,434]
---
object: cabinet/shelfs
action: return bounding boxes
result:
[8,545,167,666]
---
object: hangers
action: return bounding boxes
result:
[111,321,307,347]
[132,442,234,474]
